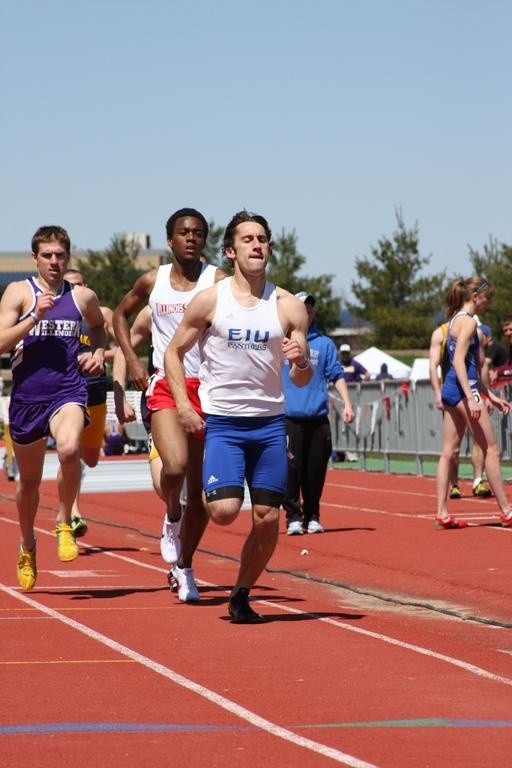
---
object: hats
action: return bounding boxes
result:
[295,291,316,304]
[480,324,492,335]
[340,344,350,353]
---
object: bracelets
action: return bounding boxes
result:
[294,364,311,370]
[30,312,40,324]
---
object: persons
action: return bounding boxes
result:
[434,274,512,529]
[325,345,369,463]
[161,210,315,626]
[375,363,393,379]
[430,296,512,497]
[112,205,233,603]
[54,269,116,535]
[282,290,355,540]
[197,252,209,266]
[0,225,110,594]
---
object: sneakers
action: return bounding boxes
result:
[70,516,87,536]
[449,485,461,498]
[308,521,324,533]
[168,563,200,602]
[287,520,304,535]
[160,503,183,562]
[473,480,491,496]
[500,511,512,528]
[16,535,36,591]
[57,523,79,562]
[434,514,467,529]
[227,587,266,623]
[348,454,358,462]
